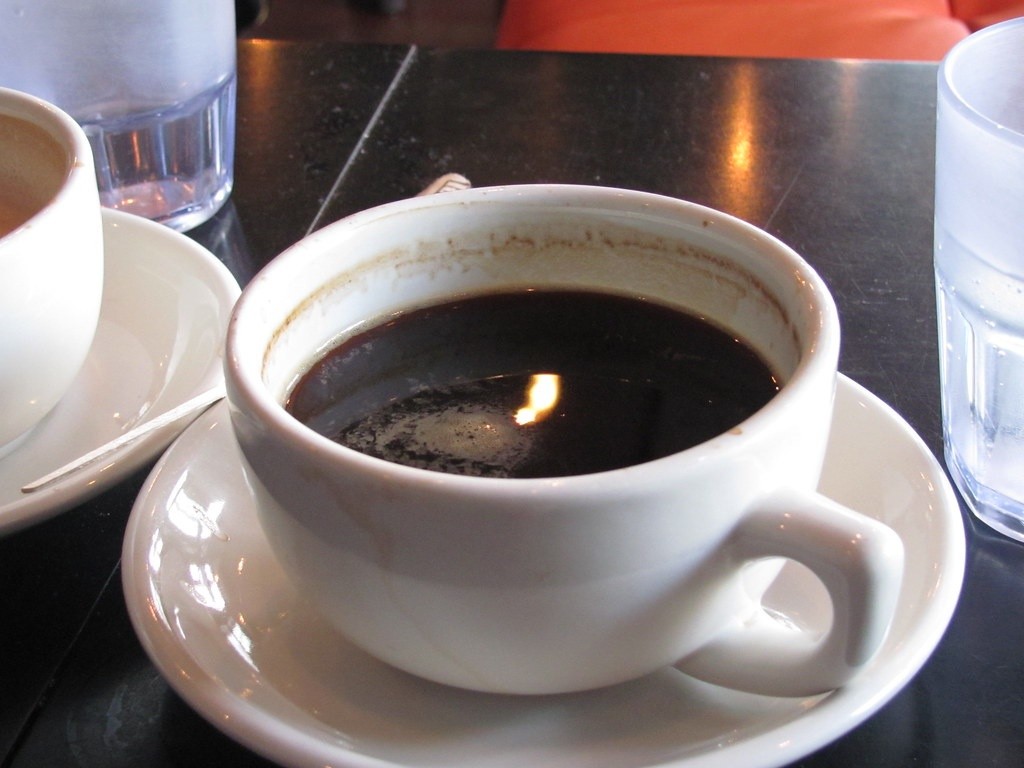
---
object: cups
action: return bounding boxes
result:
[934,18,1023,543]
[1,85,105,466]
[224,184,909,698]
[1,0,238,238]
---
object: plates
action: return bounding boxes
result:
[120,374,967,768]
[0,207,246,539]
